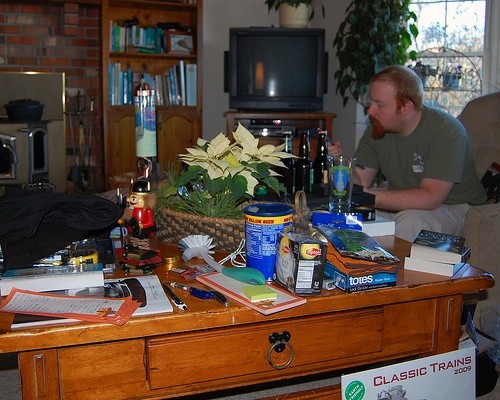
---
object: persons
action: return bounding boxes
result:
[328,65,487,243]
[124,179,155,251]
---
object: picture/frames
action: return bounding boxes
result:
[166,32,196,55]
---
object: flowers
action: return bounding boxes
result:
[165,123,294,214]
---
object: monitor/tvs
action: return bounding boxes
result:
[229,26,328,111]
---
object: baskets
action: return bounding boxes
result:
[154,201,311,253]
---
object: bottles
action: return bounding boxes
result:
[279,130,329,197]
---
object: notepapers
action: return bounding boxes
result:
[241,285,277,301]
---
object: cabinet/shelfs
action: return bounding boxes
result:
[225,113,337,158]
[99,0,203,190]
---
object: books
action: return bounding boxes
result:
[410,229,465,264]
[110,60,197,106]
[197,271,307,315]
[110,20,160,53]
[11,274,173,329]
[0,263,104,296]
[404,247,471,277]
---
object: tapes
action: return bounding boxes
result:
[70,250,98,265]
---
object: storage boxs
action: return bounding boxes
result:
[325,262,397,292]
[275,233,328,296]
[362,216,395,237]
[282,183,376,222]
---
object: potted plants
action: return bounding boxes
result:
[263,0,326,28]
[442,65,461,89]
[408,60,436,86]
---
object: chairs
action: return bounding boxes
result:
[0,192,118,368]
[405,91,500,334]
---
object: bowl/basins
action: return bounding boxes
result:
[4,103,44,122]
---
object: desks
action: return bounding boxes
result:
[0,237,497,400]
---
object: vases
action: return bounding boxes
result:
[157,201,309,252]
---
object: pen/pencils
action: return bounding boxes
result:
[162,284,187,311]
[170,282,215,300]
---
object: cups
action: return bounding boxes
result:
[327,154,358,209]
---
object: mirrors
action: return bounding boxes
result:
[0,68,68,194]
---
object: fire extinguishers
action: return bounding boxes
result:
[133,72,157,95]
